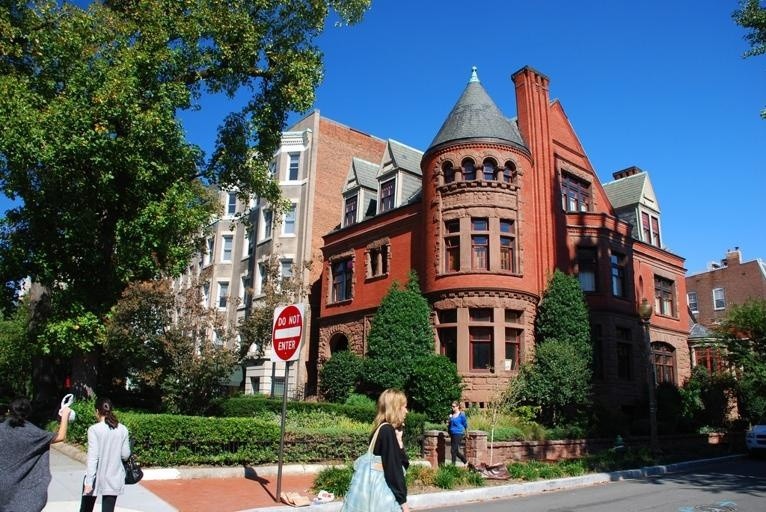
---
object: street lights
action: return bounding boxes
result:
[638,295,660,464]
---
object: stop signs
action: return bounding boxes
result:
[268,303,306,363]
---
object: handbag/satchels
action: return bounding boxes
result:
[340,422,402,512]
[121,454,143,484]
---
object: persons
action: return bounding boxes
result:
[448,399,472,467]
[369,387,412,512]
[0,399,71,511]
[78,395,131,511]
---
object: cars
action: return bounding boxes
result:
[746,412,766,458]
